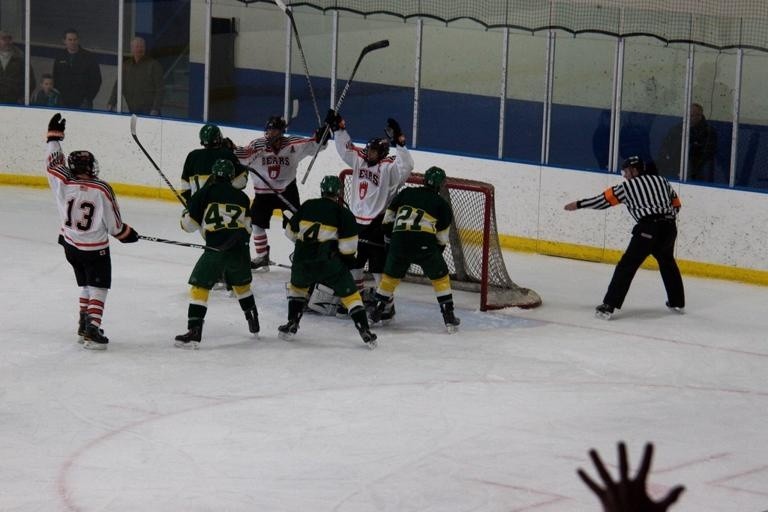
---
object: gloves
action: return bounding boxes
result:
[324,109,347,132]
[316,125,331,146]
[113,223,140,244]
[384,117,407,148]
[47,112,67,143]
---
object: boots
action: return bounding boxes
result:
[78,311,105,337]
[84,323,109,344]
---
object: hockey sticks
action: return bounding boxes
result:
[250,99,299,162]
[302,40,390,184]
[138,231,242,253]
[276,1,321,127]
[282,210,387,247]
[243,166,298,213]
[131,114,188,209]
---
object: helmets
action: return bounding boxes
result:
[67,150,99,177]
[423,166,447,188]
[265,114,287,133]
[620,155,644,171]
[366,137,390,156]
[211,159,236,177]
[320,175,343,197]
[199,124,222,146]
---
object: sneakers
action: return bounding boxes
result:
[666,301,685,309]
[595,302,616,314]
[335,288,396,323]
[249,245,270,269]
[174,323,203,343]
[442,310,461,327]
[359,328,377,343]
[277,319,298,334]
[247,315,260,334]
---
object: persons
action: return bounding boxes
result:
[106,38,166,116]
[175,109,460,343]
[52,29,101,109]
[0,29,35,105]
[30,73,62,107]
[564,155,685,315]
[45,114,138,344]
[662,104,719,182]
[577,442,683,512]
